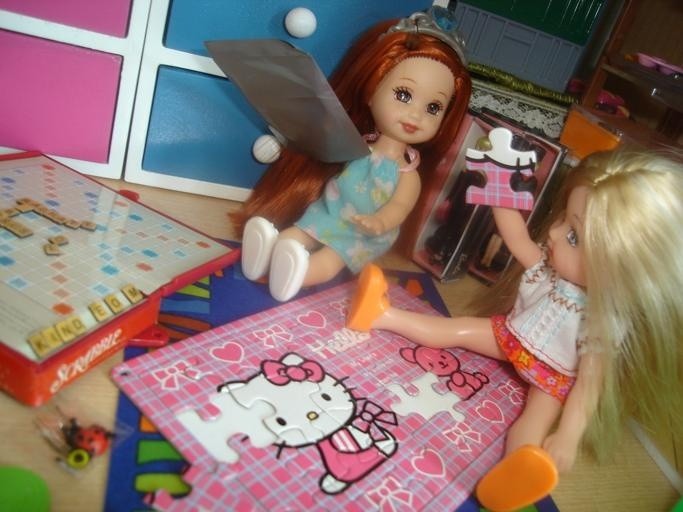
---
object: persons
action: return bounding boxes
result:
[342,148,682,511]
[229,10,477,307]
